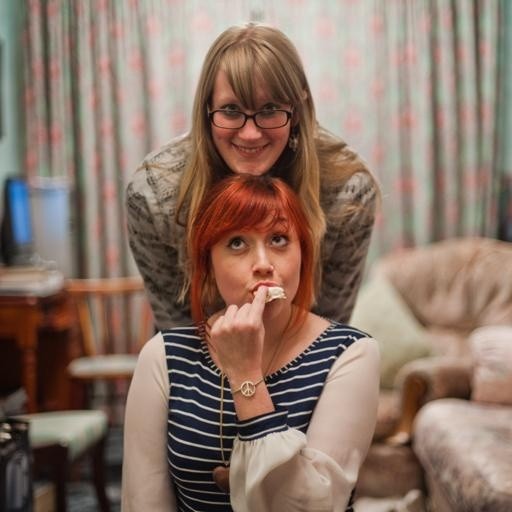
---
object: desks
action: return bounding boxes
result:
[0,267,86,416]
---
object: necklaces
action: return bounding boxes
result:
[212,304,293,494]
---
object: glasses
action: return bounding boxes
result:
[206,102,295,129]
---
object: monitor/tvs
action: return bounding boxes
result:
[0,171,35,269]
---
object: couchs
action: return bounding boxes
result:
[412,325,512,511]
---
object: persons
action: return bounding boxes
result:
[120,173,381,512]
[124,23,381,337]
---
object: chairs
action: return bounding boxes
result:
[354,237,512,496]
[63,276,158,433]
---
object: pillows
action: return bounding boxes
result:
[349,270,432,387]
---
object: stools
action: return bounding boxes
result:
[0,408,112,512]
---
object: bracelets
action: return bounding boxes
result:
[230,378,264,398]
